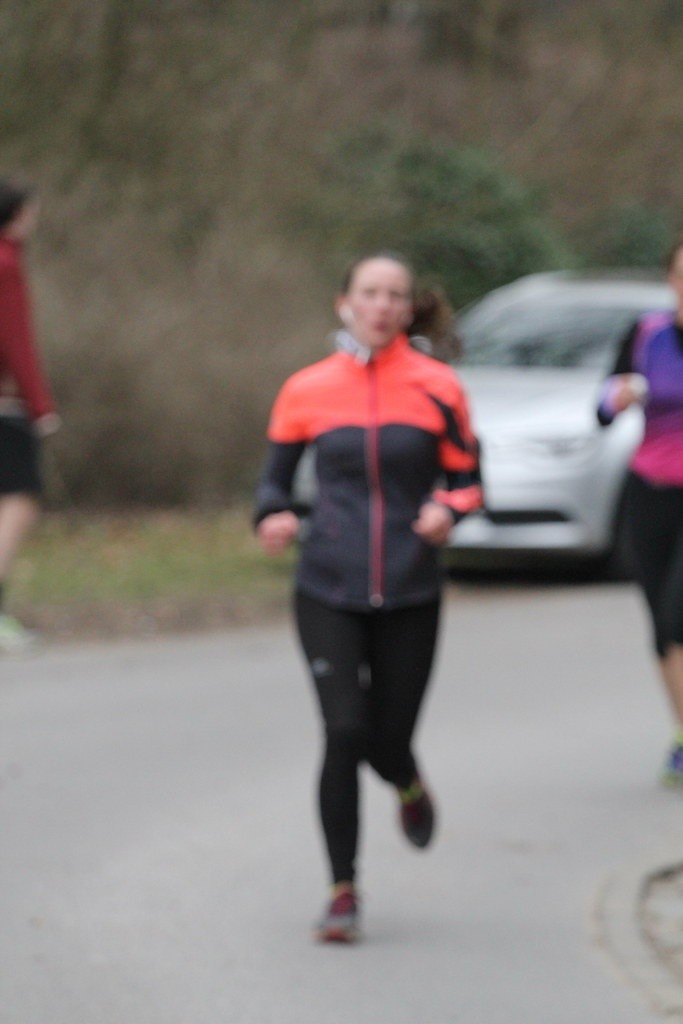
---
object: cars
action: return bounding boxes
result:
[415,265,683,583]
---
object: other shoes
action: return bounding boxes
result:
[325,894,356,943]
[396,775,434,848]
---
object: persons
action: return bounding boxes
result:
[590,239,683,791]
[0,175,62,657]
[249,254,485,945]
